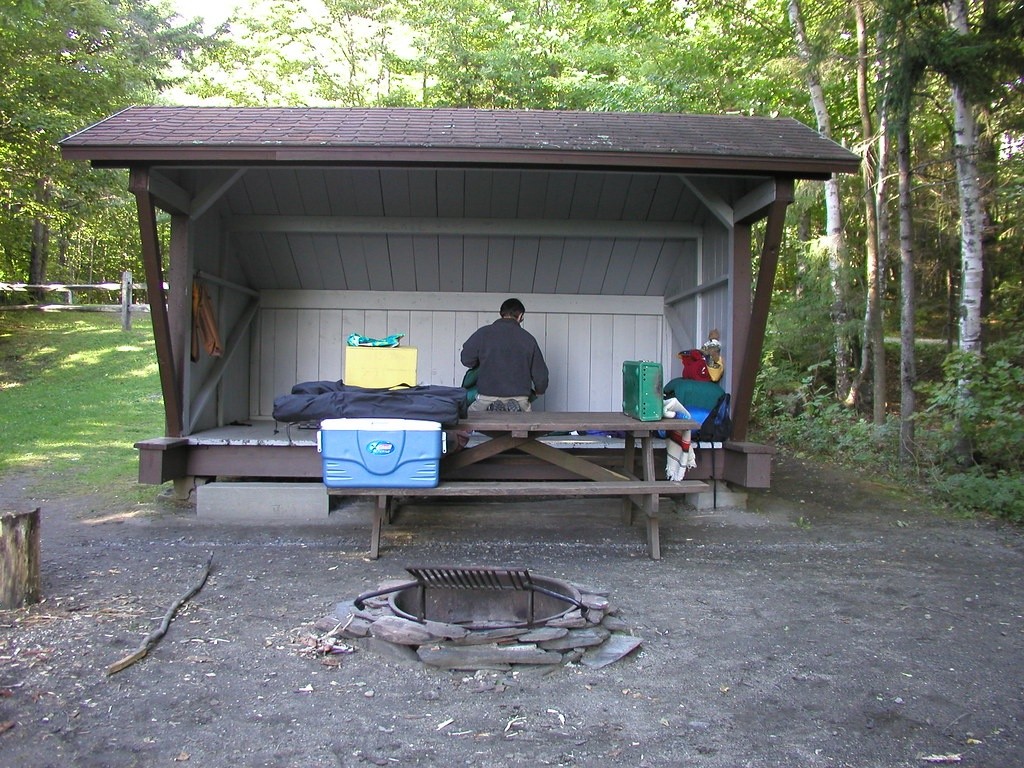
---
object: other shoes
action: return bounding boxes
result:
[487,400,506,411]
[507,398,521,412]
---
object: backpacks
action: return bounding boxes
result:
[698,393,732,441]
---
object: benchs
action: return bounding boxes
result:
[331,478,711,557]
[443,450,660,528]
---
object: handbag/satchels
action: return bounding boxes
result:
[347,331,405,348]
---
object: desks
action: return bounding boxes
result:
[368,411,701,560]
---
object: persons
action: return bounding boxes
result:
[461,298,548,413]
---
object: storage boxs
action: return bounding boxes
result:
[621,360,664,421]
[345,346,418,391]
[316,418,448,489]
